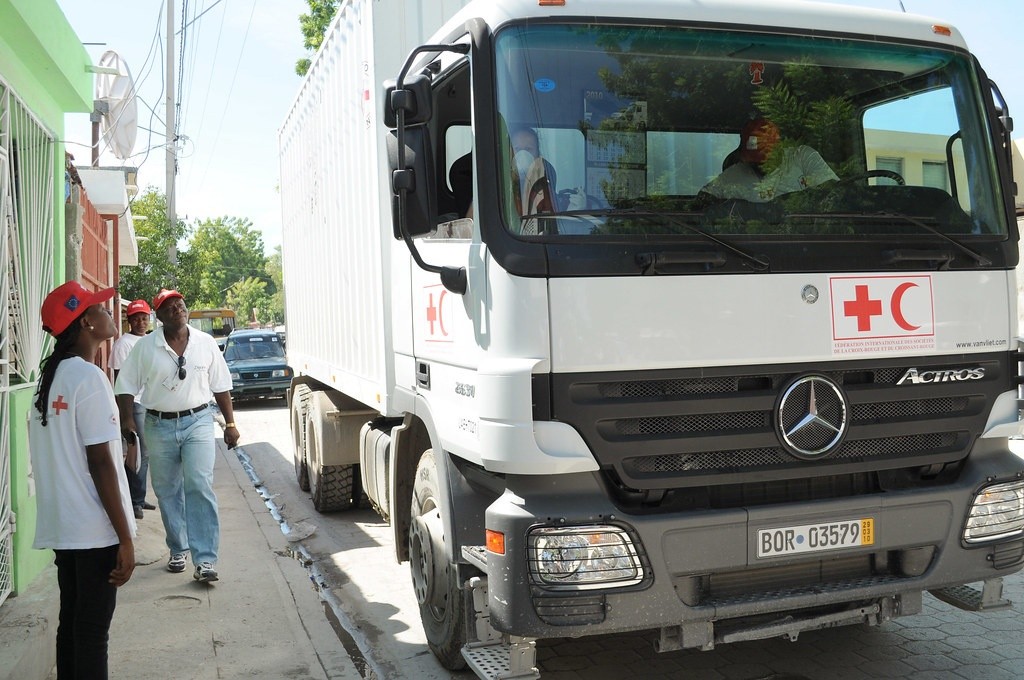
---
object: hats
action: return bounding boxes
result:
[153,288,184,311]
[737,119,777,162]
[126,300,151,316]
[41,280,115,336]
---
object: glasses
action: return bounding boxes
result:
[178,356,187,380]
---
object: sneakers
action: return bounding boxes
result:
[167,552,187,572]
[193,562,219,583]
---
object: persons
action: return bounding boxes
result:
[27,280,139,680]
[107,287,240,584]
[465,125,560,221]
[696,118,843,206]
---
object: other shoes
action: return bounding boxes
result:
[141,502,156,510]
[133,506,143,519]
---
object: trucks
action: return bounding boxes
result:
[279,1,1024,679]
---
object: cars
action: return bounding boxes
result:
[218,327,294,398]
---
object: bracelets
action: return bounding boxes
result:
[226,423,235,427]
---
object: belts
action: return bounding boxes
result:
[146,403,208,419]
